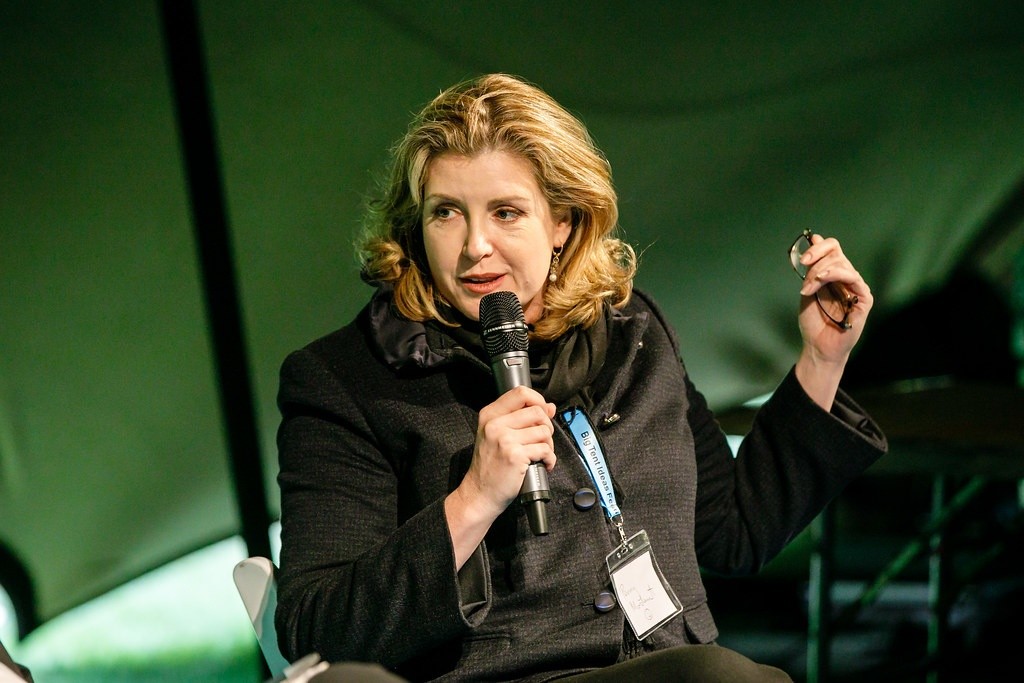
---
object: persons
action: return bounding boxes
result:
[275,68,890,683]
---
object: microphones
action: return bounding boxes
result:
[479,290,549,536]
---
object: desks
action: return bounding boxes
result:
[713,373,1024,683]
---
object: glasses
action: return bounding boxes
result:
[789,227,859,330]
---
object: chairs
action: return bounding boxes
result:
[232,556,296,683]
